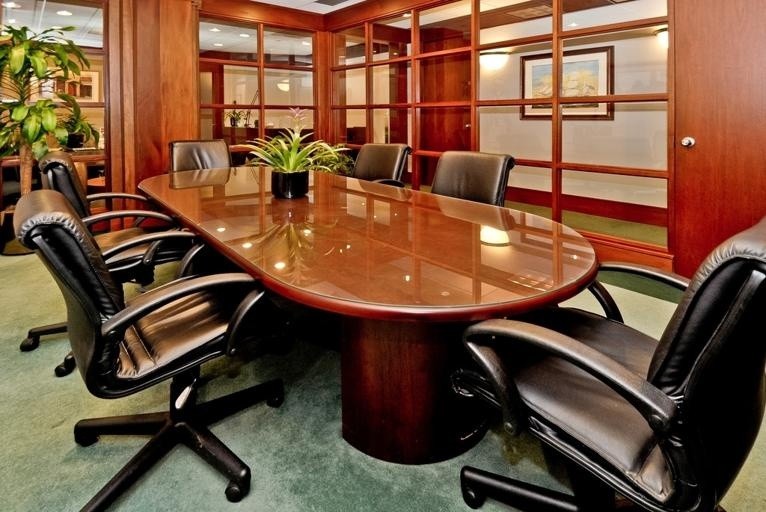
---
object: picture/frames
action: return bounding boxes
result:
[519,43,615,121]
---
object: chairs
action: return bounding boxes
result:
[355,139,412,187]
[168,139,231,177]
[449,207,766,512]
[14,190,299,512]
[429,150,516,209]
[15,150,198,380]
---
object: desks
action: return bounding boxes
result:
[138,163,601,466]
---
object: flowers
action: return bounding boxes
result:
[240,107,360,178]
[224,110,244,127]
